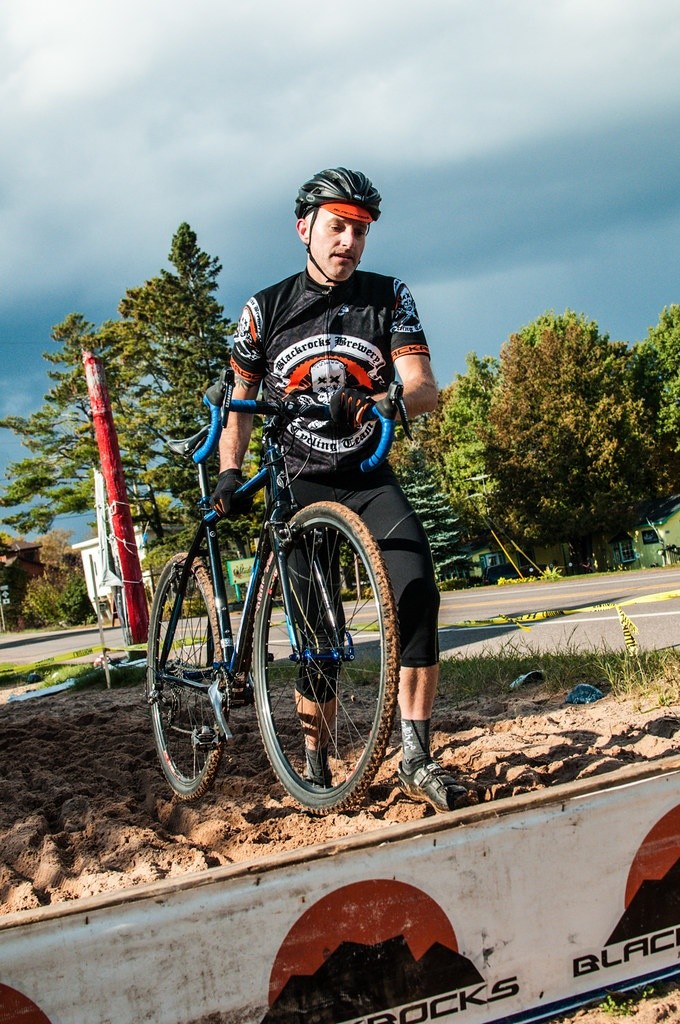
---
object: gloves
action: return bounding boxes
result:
[210,469,253,522]
[330,388,378,428]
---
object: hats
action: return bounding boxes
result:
[320,203,374,224]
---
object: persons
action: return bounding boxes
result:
[210,166,467,813]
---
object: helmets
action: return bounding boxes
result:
[293,166,382,222]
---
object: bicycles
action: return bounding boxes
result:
[141,367,413,816]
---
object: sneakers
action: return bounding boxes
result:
[397,759,468,814]
[303,769,332,788]
